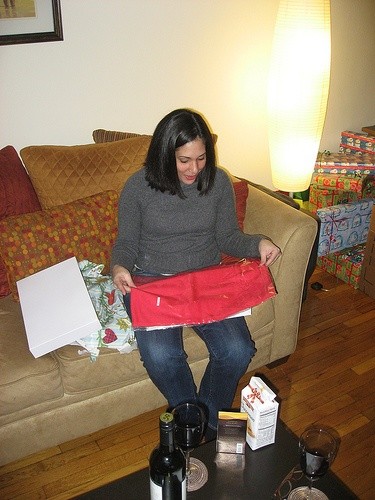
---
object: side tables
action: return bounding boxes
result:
[291,197,323,303]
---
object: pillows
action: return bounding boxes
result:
[0,145,42,299]
[0,189,119,304]
[92,129,141,143]
[233,179,248,232]
[19,134,152,210]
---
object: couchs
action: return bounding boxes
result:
[0,129,318,469]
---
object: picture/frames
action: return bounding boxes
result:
[0,0,64,47]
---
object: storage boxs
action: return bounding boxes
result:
[309,130,375,301]
[240,376,279,451]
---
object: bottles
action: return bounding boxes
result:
[148,413,186,500]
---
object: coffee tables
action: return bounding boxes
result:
[68,420,360,500]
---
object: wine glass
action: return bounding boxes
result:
[293,428,336,500]
[170,403,204,485]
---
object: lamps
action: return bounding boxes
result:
[266,0,332,201]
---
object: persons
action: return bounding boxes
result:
[110,108,282,450]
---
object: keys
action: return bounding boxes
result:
[312,282,328,292]
